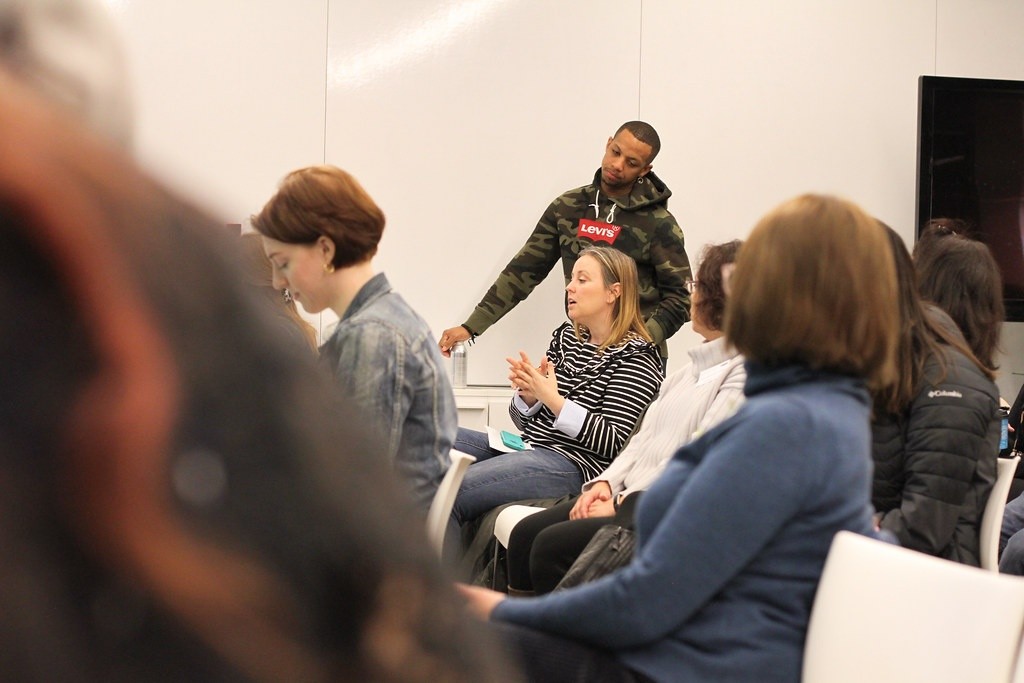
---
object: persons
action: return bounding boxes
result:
[0,0,1024,683]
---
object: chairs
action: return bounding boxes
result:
[801,531,1023,683]
[978,385,1023,575]
[426,446,476,562]
[490,504,551,589]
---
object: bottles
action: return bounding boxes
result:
[448,339,467,389]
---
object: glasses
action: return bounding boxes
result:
[686,281,703,294]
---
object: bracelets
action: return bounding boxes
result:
[613,493,623,512]
[461,323,476,346]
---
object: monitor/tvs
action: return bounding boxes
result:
[914,76,1024,323]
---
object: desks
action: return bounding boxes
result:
[451,382,540,452]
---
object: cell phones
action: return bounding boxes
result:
[500,431,525,451]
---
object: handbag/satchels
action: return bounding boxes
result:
[548,524,637,593]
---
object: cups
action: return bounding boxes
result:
[998,407,1012,458]
